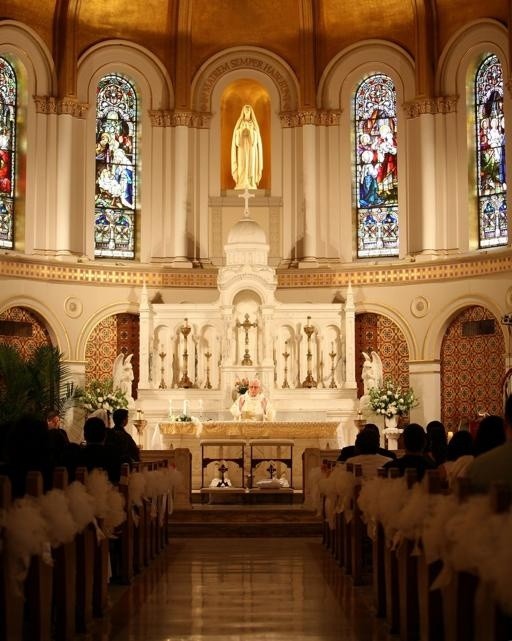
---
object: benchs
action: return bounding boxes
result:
[321,459,512,639]
[1,460,172,641]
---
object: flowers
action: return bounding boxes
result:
[366,379,420,417]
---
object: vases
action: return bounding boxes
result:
[384,414,400,428]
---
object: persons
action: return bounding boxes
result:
[0,408,143,505]
[230,105,263,190]
[479,116,505,187]
[357,133,384,207]
[374,125,398,193]
[118,362,135,400]
[1,106,134,210]
[360,360,380,396]
[229,376,275,423]
[335,389,511,515]
